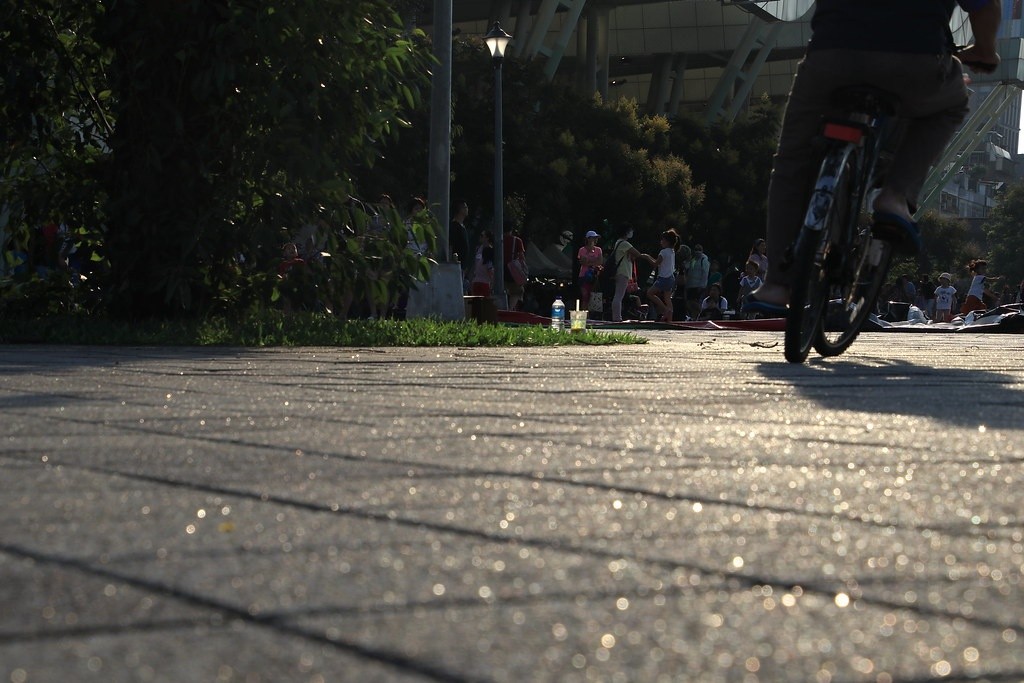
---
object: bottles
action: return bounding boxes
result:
[551,295,565,333]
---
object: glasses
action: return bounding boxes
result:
[479,233,485,237]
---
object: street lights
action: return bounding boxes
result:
[482,19,513,318]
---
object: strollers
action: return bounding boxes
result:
[592,265,650,321]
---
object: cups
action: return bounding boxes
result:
[569,310,589,335]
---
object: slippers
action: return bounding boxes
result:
[741,292,784,313]
[876,213,922,256]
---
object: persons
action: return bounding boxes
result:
[1,176,1024,322]
[749,0,1000,307]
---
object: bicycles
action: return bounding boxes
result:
[782,45,998,365]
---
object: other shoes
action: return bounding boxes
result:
[662,308,671,320]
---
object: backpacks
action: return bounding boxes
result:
[604,240,625,276]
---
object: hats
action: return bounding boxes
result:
[938,273,951,282]
[586,231,601,238]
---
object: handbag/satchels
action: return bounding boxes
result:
[588,292,603,313]
[508,237,529,286]
[698,308,721,321]
